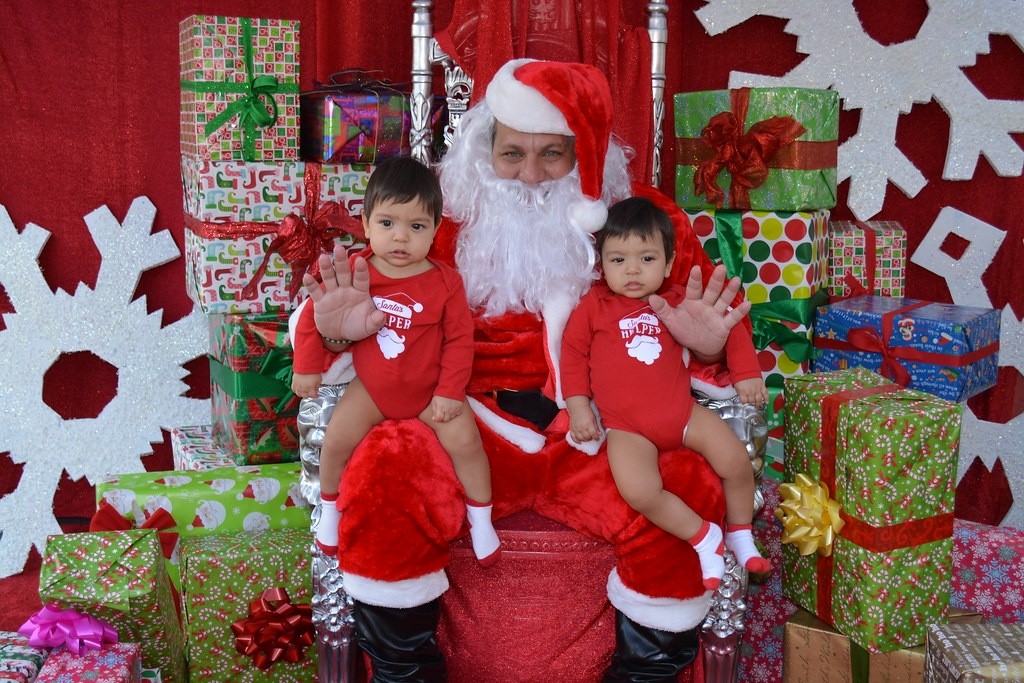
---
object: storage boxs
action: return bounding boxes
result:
[0,15,1024,683]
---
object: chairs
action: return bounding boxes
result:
[288,0,769,683]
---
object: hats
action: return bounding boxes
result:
[485,58,614,202]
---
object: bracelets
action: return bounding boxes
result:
[323,336,352,344]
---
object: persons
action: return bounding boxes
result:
[290,58,753,683]
[561,196,771,589]
[294,157,502,567]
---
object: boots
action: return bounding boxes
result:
[339,569,451,683]
[603,567,715,683]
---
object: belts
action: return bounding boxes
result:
[486,387,562,430]
